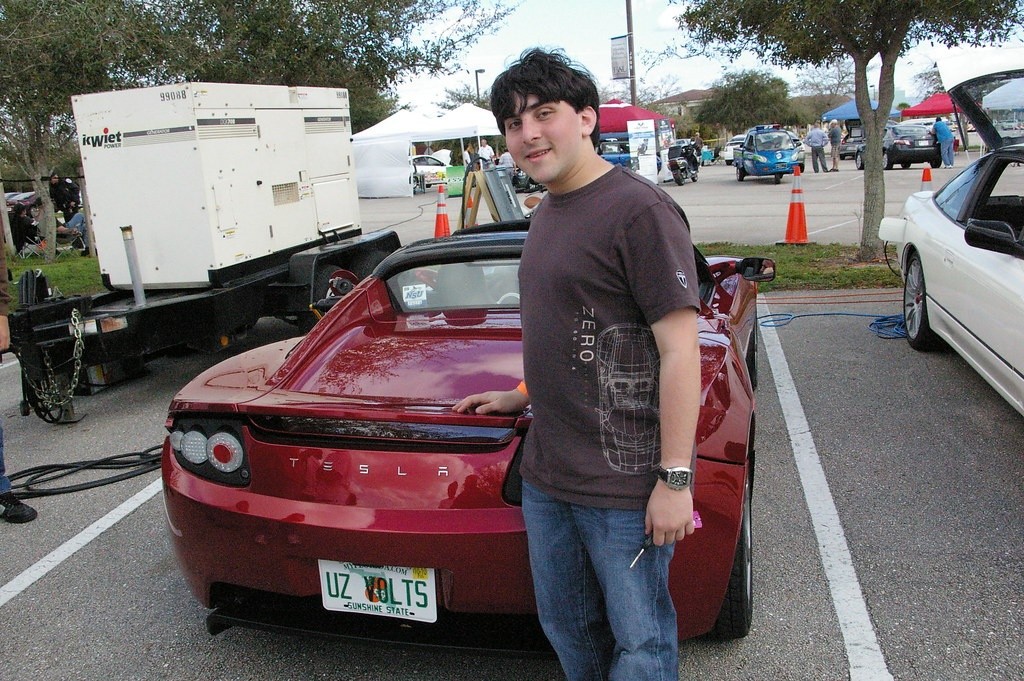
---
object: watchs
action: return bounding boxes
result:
[657,465,694,491]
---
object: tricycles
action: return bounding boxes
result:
[732,123,799,184]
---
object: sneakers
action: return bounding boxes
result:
[0,492,37,523]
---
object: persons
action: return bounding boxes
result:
[802,123,829,173]
[464,138,495,173]
[685,132,704,167]
[641,139,648,151]
[453,34,700,681]
[48,173,84,250]
[64,212,89,257]
[771,138,781,150]
[4,202,43,254]
[826,119,841,172]
[931,117,954,169]
[0,208,38,523]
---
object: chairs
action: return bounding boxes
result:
[56,232,83,259]
[16,235,45,260]
[702,147,722,165]
[437,263,487,305]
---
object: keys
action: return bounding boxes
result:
[629,532,653,570]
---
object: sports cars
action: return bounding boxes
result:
[159,220,781,644]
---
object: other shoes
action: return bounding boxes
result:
[830,168,838,172]
[944,165,954,170]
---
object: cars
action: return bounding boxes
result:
[876,40,1024,426]
[596,141,662,177]
[837,115,974,171]
[785,130,806,173]
[722,135,747,166]
[408,154,455,188]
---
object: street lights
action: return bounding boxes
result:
[474,68,487,106]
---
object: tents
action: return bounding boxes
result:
[599,97,677,140]
[350,102,503,170]
[980,77,1024,158]
[821,98,901,132]
[900,92,982,158]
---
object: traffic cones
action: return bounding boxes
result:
[919,166,934,194]
[462,195,479,229]
[775,167,819,246]
[434,185,452,239]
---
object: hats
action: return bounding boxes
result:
[830,120,837,125]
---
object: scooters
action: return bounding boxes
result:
[665,135,702,186]
